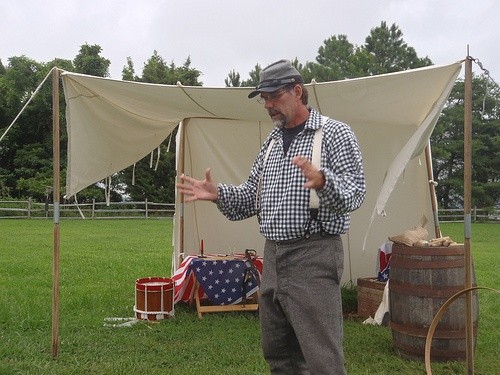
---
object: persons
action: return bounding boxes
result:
[176,59,368,375]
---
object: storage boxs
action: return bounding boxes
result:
[358,278,387,319]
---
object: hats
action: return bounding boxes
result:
[248,59,304,98]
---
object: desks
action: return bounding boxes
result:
[187,254,263,320]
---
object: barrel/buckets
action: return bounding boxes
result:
[388,242,480,363]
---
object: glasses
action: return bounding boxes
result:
[257,84,296,104]
